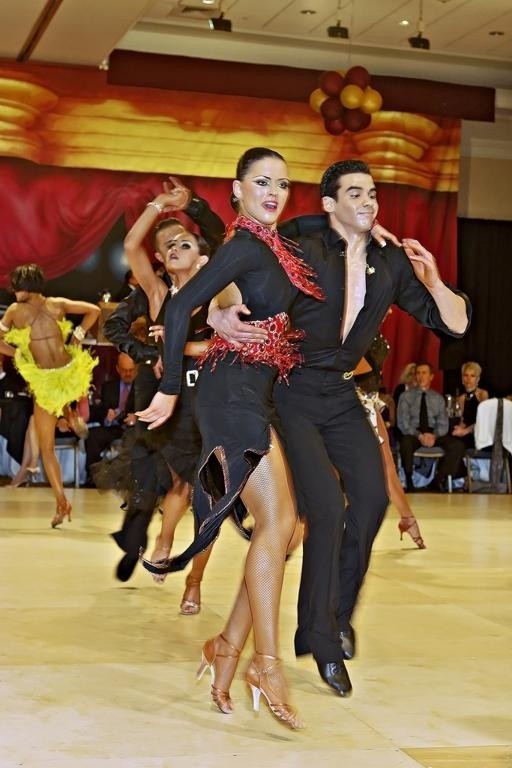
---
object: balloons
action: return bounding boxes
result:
[308,65,384,136]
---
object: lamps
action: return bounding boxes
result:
[208,10,233,32]
[409,30,431,50]
[326,19,350,39]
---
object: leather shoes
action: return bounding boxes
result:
[312,630,354,691]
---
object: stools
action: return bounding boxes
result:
[462,447,512,494]
[54,435,82,489]
[412,446,445,490]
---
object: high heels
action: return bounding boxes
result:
[5,466,40,488]
[399,520,425,548]
[64,401,88,439]
[52,500,72,528]
[196,639,305,731]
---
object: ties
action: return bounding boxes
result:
[420,392,429,434]
[119,383,128,412]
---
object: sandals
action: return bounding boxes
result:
[151,536,200,615]
